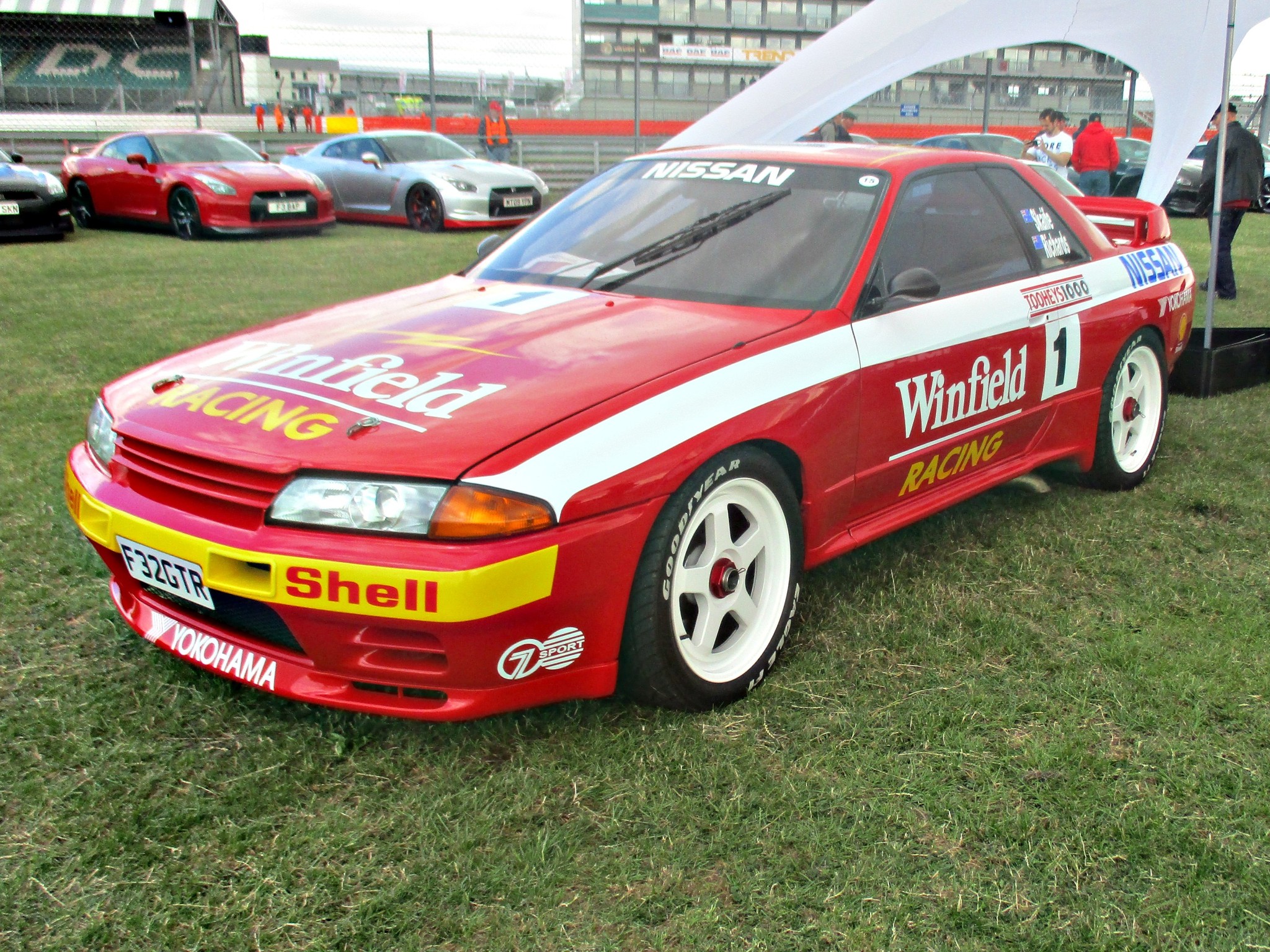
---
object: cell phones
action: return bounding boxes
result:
[1032,141,1038,146]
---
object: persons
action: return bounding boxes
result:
[285,103,298,132]
[740,76,756,92]
[274,104,285,132]
[303,105,313,134]
[256,103,265,133]
[819,110,858,143]
[1020,108,1074,180]
[1194,102,1264,301]
[477,101,513,164]
[1071,112,1119,197]
[1022,111,1088,168]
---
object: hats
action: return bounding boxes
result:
[1215,102,1237,114]
[488,100,502,112]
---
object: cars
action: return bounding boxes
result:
[1102,138,1208,218]
[799,133,876,146]
[0,147,71,240]
[906,130,1086,207]
[58,129,336,237]
[1183,141,1269,211]
[280,130,549,231]
[70,144,1192,720]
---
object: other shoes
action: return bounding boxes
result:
[1200,279,1236,300]
